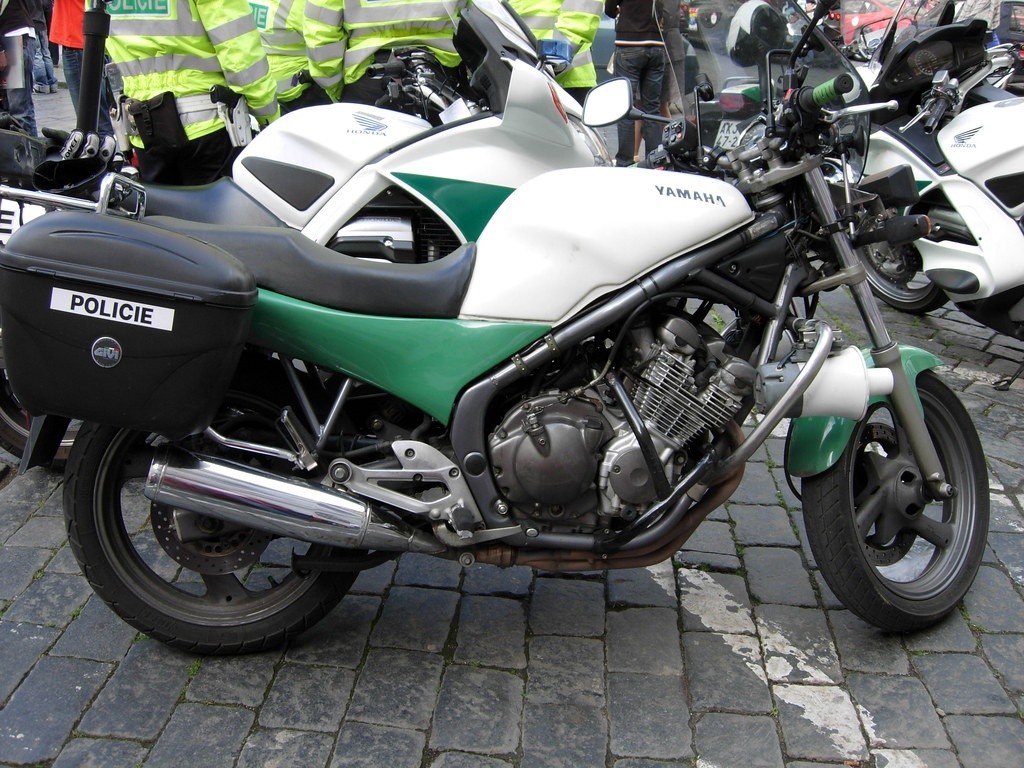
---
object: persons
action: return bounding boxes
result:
[597,0,684,167]
[0,0,605,185]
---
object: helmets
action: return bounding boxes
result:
[725,0,787,68]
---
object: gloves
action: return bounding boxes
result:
[31,125,115,192]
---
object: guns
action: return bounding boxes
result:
[209,82,243,124]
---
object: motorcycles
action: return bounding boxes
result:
[0,0,1024,655]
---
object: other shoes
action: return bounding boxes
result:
[612,155,639,166]
[50,82,58,92]
[33,83,50,94]
